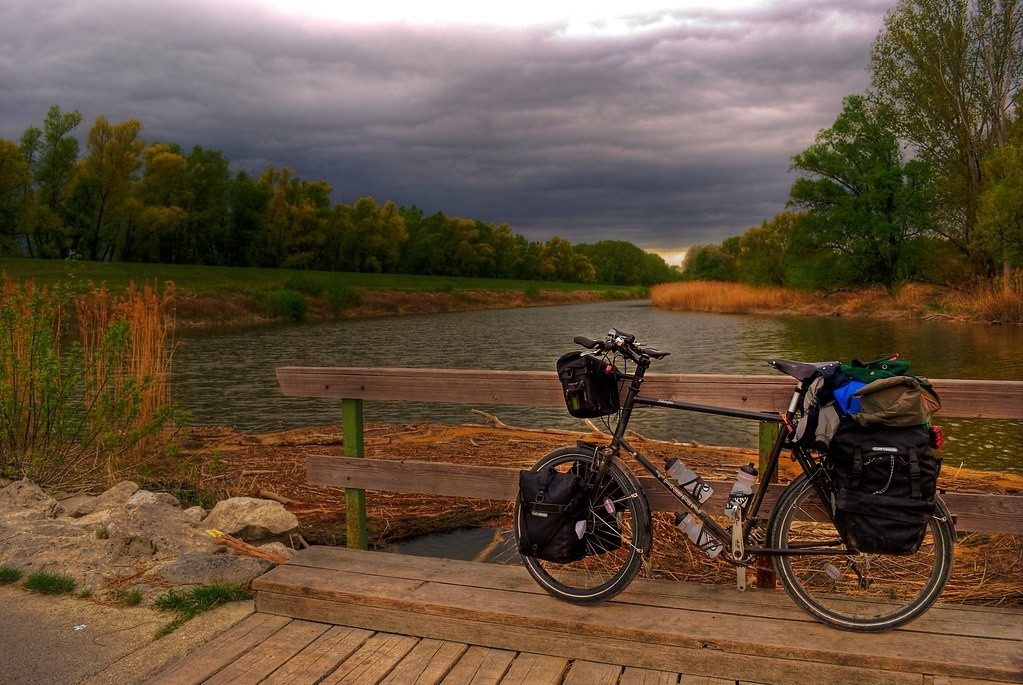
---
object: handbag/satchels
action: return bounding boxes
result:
[821,418,943,556]
[516,466,589,564]
[847,376,943,427]
[566,460,624,556]
[839,352,933,388]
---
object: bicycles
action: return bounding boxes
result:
[514,327,958,633]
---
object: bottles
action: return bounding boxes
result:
[664,456,714,504]
[724,462,758,518]
[673,511,724,560]
